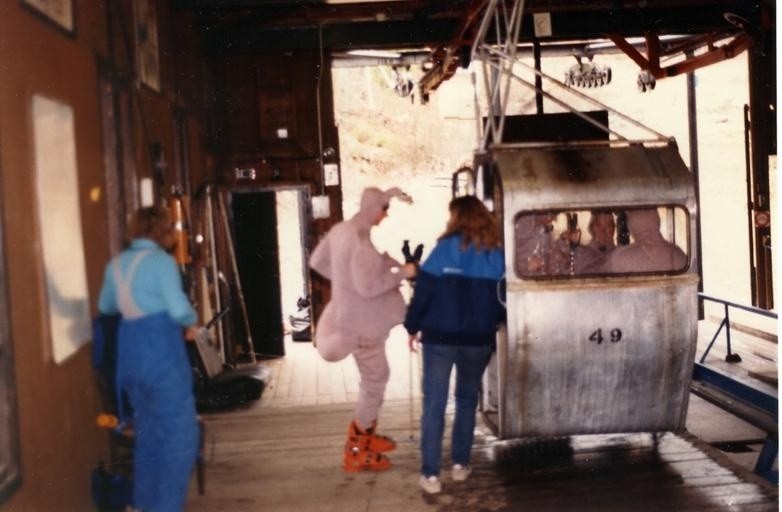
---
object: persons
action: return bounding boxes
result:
[514,206,687,275]
[309,186,416,466]
[404,195,505,493]
[90,204,203,511]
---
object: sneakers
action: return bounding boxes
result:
[341,436,392,471]
[417,473,441,493]
[344,420,398,453]
[452,463,472,480]
